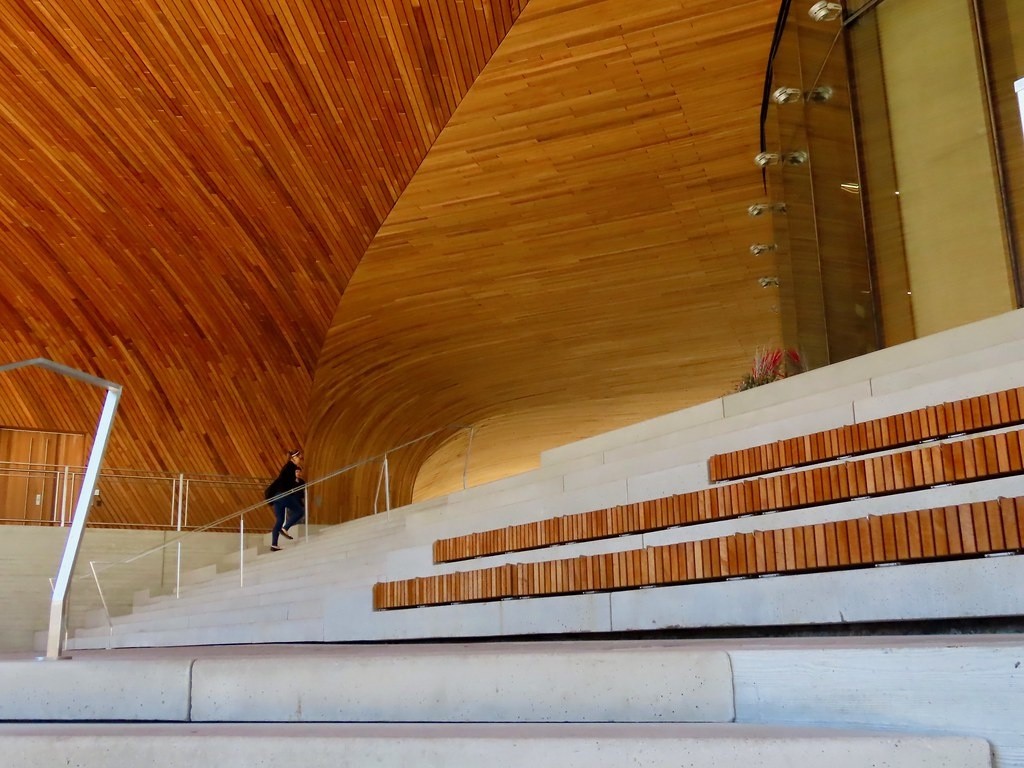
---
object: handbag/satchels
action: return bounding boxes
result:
[265,474,284,506]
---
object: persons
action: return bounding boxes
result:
[264,450,306,551]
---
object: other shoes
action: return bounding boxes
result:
[271,545,280,551]
[280,528,293,539]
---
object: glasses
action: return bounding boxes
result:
[298,455,304,461]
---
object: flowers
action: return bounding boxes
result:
[736,343,785,392]
[785,345,808,376]
